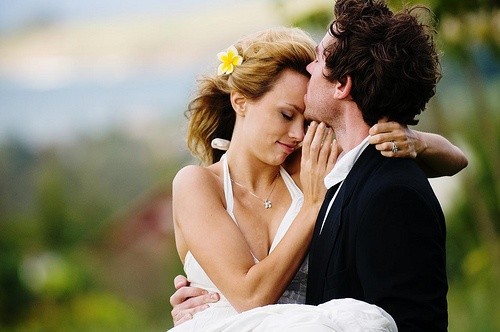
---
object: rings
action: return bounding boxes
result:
[392,142,398,152]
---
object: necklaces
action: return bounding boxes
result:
[231,173,280,209]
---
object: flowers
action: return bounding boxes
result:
[217,46,243,76]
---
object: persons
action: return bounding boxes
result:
[171,26,469,332]
[169,0,449,332]
